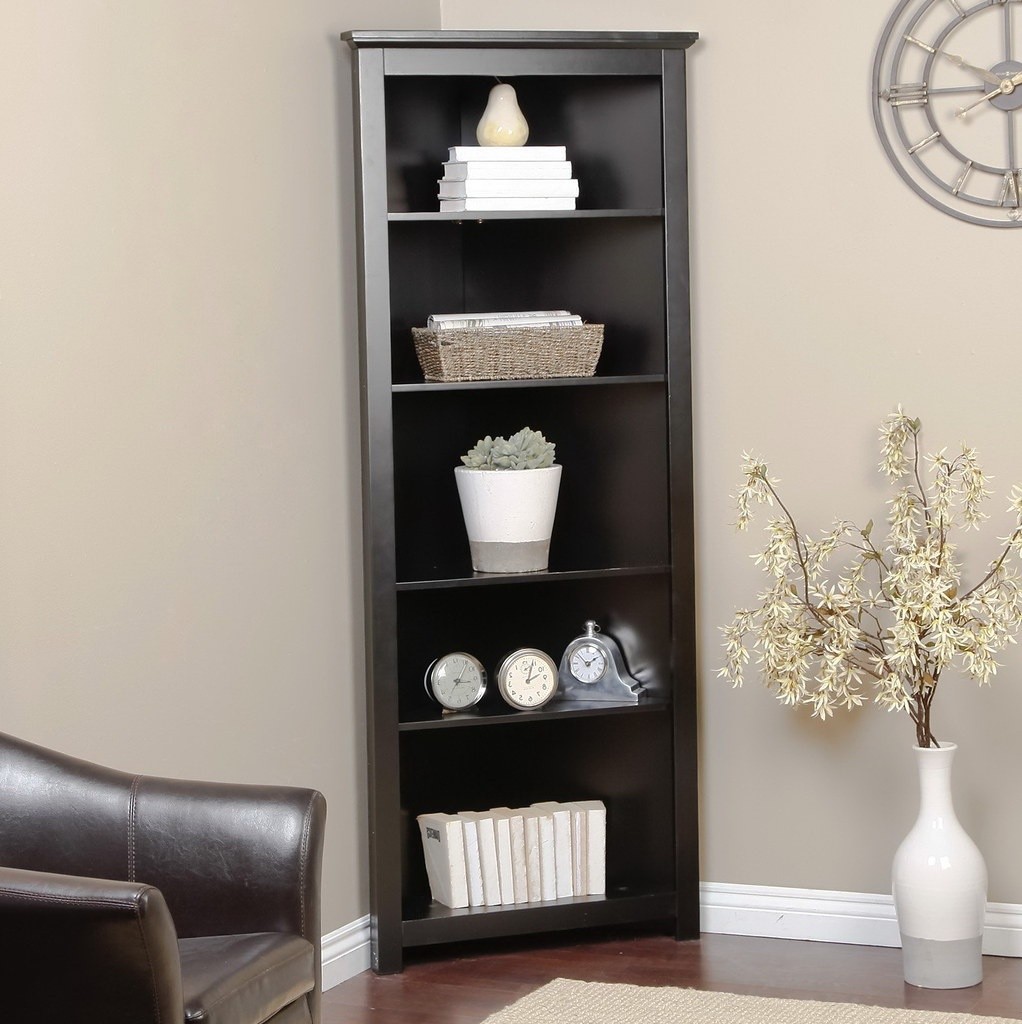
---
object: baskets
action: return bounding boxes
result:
[412,323,605,380]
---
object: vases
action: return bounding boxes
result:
[885,735,990,990]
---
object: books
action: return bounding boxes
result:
[572,800,606,895]
[530,801,573,898]
[458,811,501,906]
[450,814,484,907]
[446,146,566,162]
[441,161,572,180]
[416,813,469,909]
[520,808,556,901]
[437,179,579,197]
[513,809,541,903]
[489,807,528,904]
[560,802,586,896]
[440,198,575,212]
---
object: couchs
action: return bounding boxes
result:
[0,729,328,1024]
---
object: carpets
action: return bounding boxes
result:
[473,976,1019,1023]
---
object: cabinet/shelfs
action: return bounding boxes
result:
[338,26,724,973]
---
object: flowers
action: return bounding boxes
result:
[707,407,1022,748]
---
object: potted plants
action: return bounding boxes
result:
[455,426,563,576]
